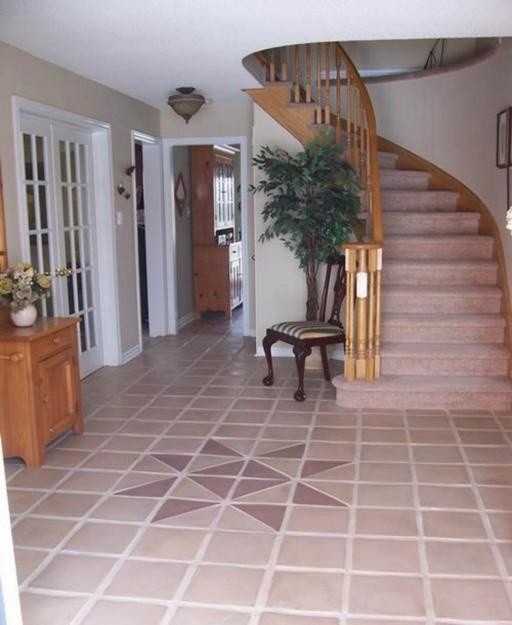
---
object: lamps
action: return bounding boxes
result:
[167,87,205,123]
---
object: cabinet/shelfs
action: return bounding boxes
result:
[189,143,244,320]
[0,317,84,469]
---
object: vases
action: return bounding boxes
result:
[10,304,37,326]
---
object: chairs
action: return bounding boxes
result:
[262,254,346,401]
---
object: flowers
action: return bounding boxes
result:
[0,263,76,314]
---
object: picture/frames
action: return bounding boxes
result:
[496,109,510,169]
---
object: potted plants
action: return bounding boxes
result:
[248,130,364,370]
